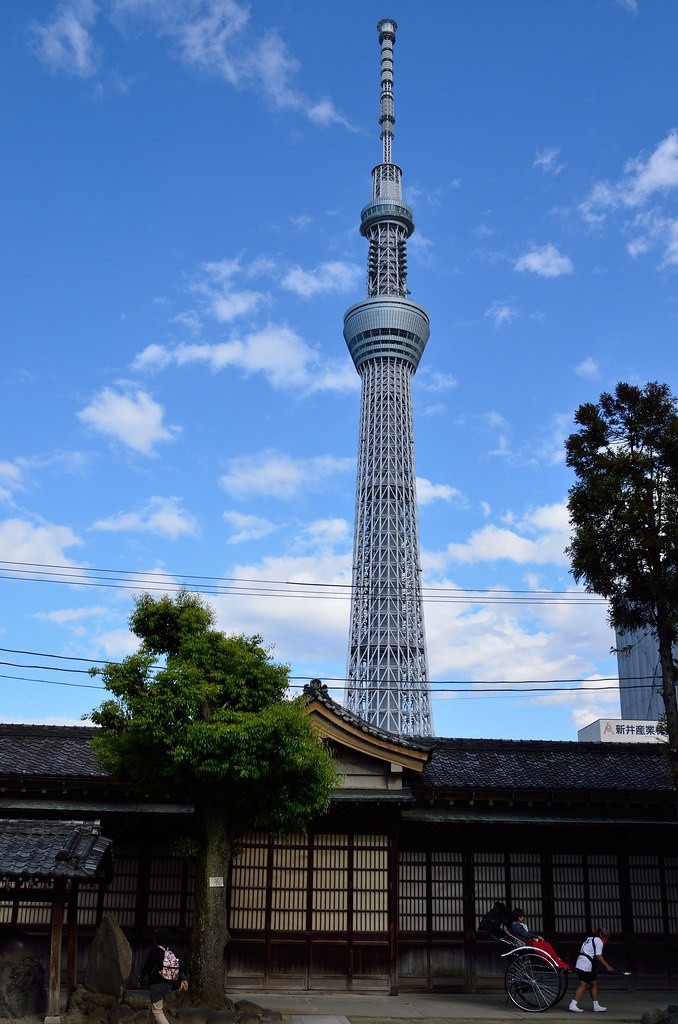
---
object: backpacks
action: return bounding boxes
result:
[157,945,180,981]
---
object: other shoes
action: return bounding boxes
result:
[593,1001,607,1012]
[569,999,584,1012]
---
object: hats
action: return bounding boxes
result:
[597,926,609,944]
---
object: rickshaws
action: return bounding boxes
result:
[477,900,632,1012]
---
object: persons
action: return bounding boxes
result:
[510,907,572,971]
[137,927,189,1024]
[568,927,615,1013]
